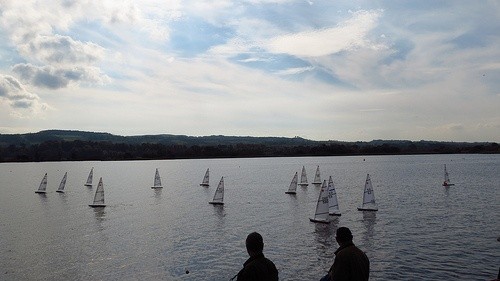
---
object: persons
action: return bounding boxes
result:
[236,232,279,281]
[319,227,370,281]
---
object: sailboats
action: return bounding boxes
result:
[88,176,107,208]
[208,175,225,205]
[308,180,331,224]
[312,163,323,184]
[298,166,310,186]
[284,171,299,194]
[55,171,68,193]
[357,173,379,211]
[84,166,95,186]
[34,172,48,194]
[327,176,342,216]
[199,168,210,187]
[441,164,455,186]
[151,167,164,189]
